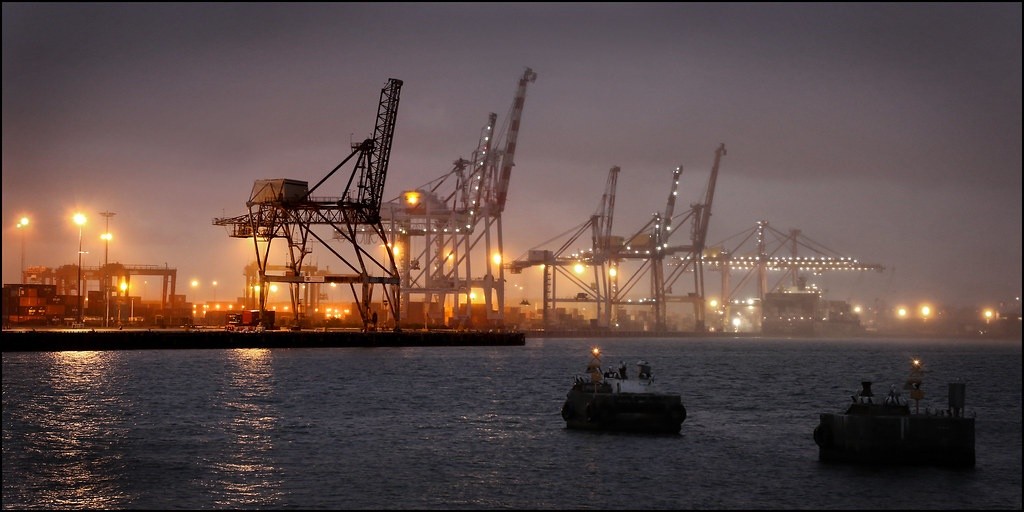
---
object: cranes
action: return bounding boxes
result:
[661,142,729,328]
[669,222,887,331]
[385,68,536,330]
[504,164,623,332]
[210,75,404,330]
[610,164,685,336]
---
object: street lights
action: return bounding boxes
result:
[72,212,87,324]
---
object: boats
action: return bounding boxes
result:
[561,369,689,438]
[813,375,978,475]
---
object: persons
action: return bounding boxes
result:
[618,359,628,379]
[604,365,617,379]
